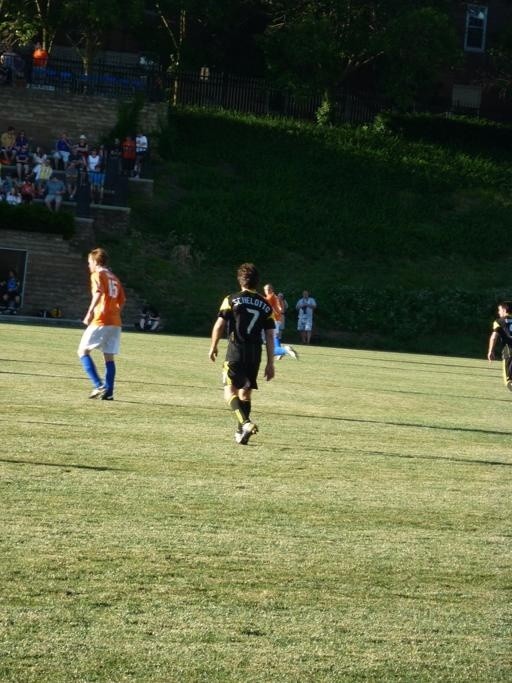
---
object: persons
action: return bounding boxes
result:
[137,302,161,332]
[0,124,151,216]
[293,286,318,345]
[29,39,50,85]
[276,291,290,344]
[261,281,300,360]
[485,299,512,395]
[0,267,25,311]
[74,245,129,403]
[208,261,276,446]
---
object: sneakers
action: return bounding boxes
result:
[235,422,258,444]
[285,345,298,361]
[89,385,113,401]
